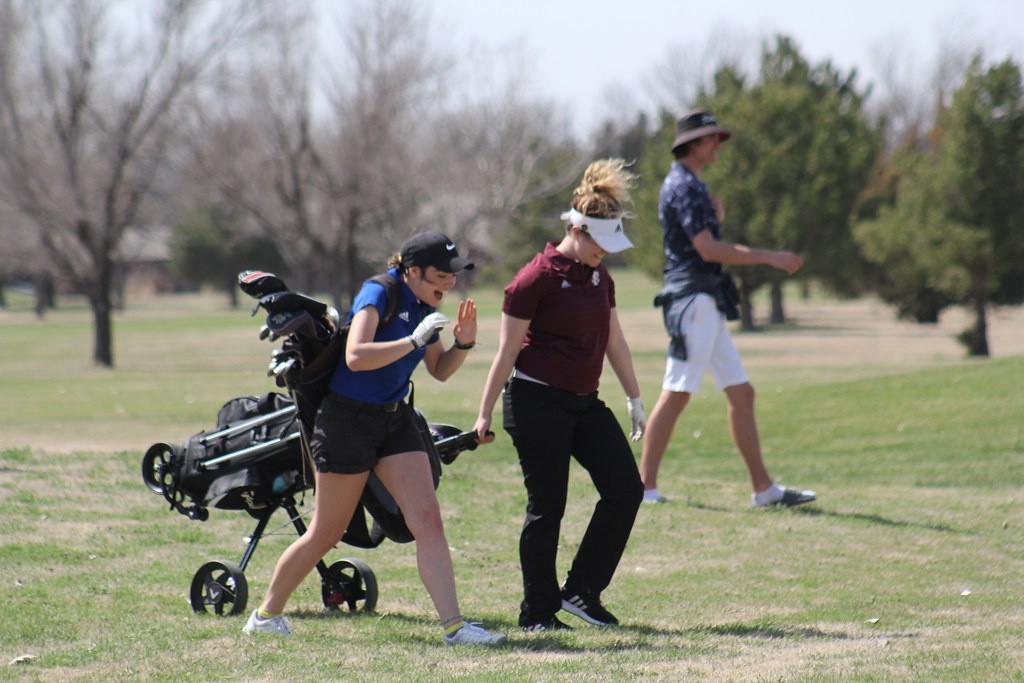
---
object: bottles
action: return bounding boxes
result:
[272,468,299,494]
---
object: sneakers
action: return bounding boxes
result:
[242,609,292,645]
[517,610,574,634]
[642,488,670,506]
[558,580,620,629]
[749,484,817,510]
[441,622,506,651]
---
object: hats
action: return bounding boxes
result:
[669,108,730,155]
[399,229,476,273]
[559,207,634,256]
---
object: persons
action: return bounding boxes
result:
[632,104,818,507]
[473,156,649,634]
[243,233,511,653]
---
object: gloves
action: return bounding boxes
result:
[626,395,646,443]
[410,311,451,349]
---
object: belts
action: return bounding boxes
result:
[328,392,399,414]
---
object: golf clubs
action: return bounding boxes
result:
[258,324,306,388]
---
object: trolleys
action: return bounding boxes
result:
[140,269,497,617]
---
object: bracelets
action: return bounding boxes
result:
[454,338,475,351]
